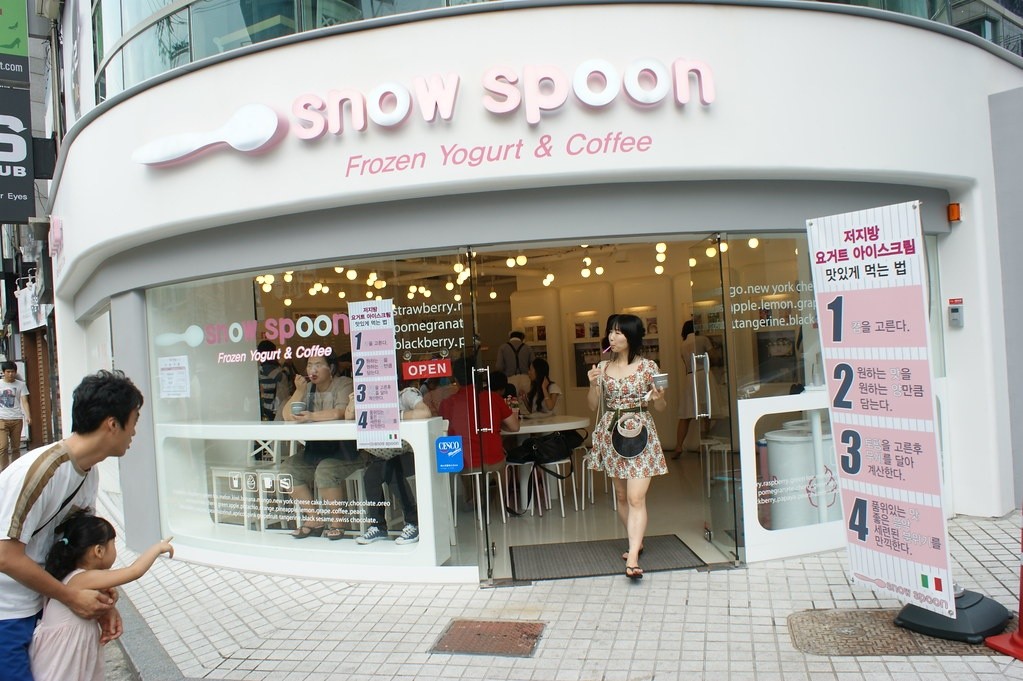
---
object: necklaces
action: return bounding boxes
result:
[317,379,333,393]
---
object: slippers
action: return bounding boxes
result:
[626,567,643,579]
[707,474,716,484]
[671,450,682,460]
[623,545,644,562]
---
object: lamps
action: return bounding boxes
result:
[26,267,38,291]
[16,276,37,294]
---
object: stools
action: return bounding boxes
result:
[702,435,743,503]
[454,447,611,529]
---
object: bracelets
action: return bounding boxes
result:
[400,411,404,420]
[544,397,550,401]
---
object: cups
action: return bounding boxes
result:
[291,402,306,415]
[652,374,669,390]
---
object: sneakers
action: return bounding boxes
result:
[355,525,389,545]
[394,525,419,545]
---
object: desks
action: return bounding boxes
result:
[501,415,591,510]
[160,417,449,566]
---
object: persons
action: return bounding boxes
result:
[254,330,572,544]
[520,358,562,421]
[588,314,668,579]
[0,361,32,474]
[673,320,722,459]
[494,330,535,375]
[283,348,366,538]
[345,386,432,544]
[33,509,174,681]
[436,362,520,519]
[0,368,143,681]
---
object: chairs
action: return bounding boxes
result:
[211,421,448,533]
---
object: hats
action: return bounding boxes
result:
[611,414,649,459]
[508,328,527,339]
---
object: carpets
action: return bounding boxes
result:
[510,534,705,581]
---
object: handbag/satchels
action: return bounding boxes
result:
[506,428,587,515]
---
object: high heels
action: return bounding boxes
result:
[290,524,325,539]
[328,526,346,540]
